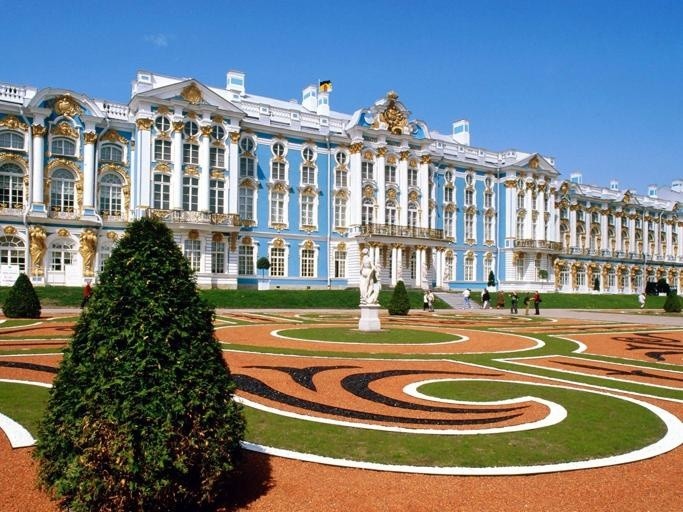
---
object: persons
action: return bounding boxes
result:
[638,292,646,309]
[360,247,383,305]
[480,288,493,309]
[423,289,435,312]
[496,290,540,316]
[463,288,473,310]
[79,279,92,310]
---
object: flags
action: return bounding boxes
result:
[319,80,332,92]
